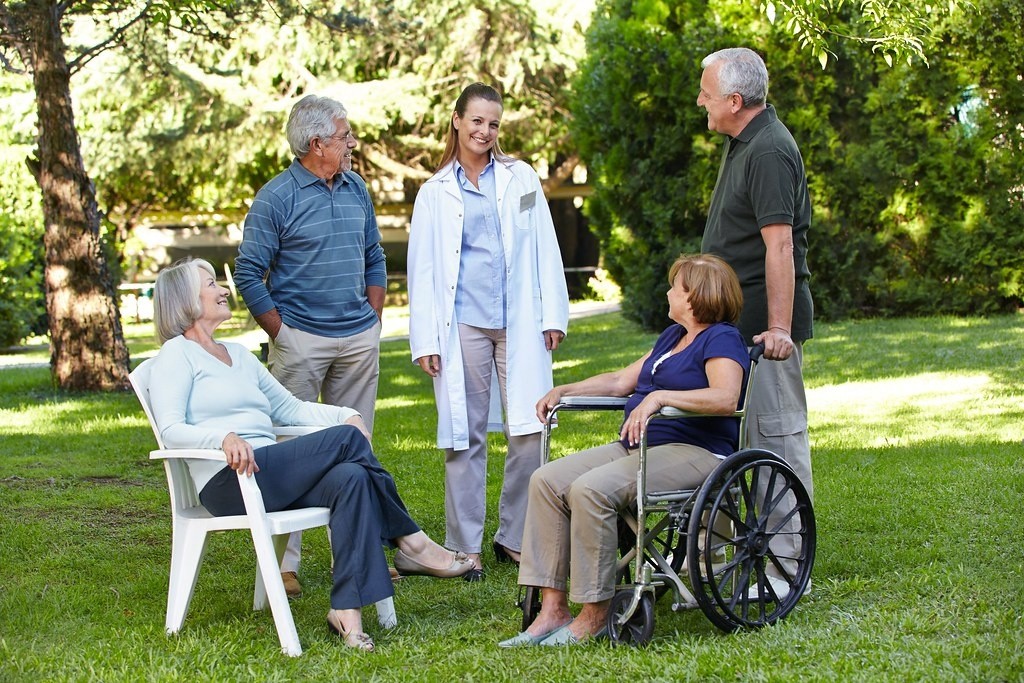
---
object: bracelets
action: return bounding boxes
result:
[767,327,791,337]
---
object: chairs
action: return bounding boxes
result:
[128,357,397,658]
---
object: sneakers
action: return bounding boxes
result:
[645,553,727,579]
[739,574,811,599]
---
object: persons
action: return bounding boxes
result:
[144,257,477,652]
[406,82,569,584]
[640,47,816,603]
[497,251,750,654]
[232,93,402,593]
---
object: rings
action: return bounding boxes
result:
[634,420,640,424]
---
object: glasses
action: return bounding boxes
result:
[325,128,352,142]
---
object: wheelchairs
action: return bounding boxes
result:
[511,340,818,653]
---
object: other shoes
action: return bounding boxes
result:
[540,626,608,647]
[498,620,573,647]
[280,572,302,595]
[389,567,399,580]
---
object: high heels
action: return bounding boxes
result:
[461,569,486,583]
[327,608,375,652]
[493,542,519,567]
[394,547,476,578]
